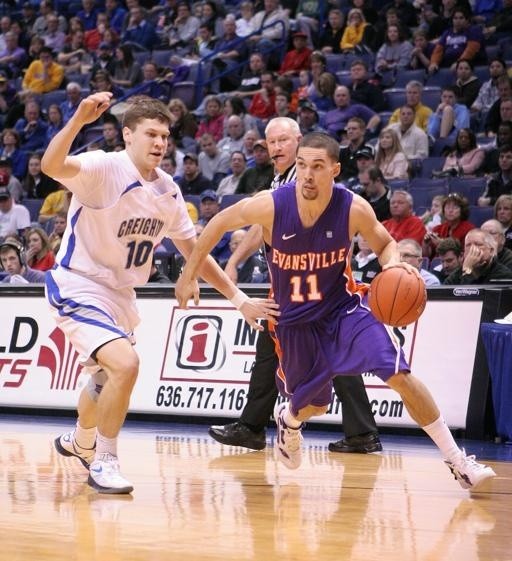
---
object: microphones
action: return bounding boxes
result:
[268,156,278,162]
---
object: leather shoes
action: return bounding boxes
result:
[329,434,382,453]
[207,421,267,449]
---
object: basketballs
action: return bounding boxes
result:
[368,267,428,326]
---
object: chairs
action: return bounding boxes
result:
[0,0,512,282]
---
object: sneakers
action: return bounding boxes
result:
[273,402,304,471]
[445,448,495,489]
[88,459,133,495]
[54,433,95,468]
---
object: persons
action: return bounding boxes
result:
[174,132,498,492]
[41,90,283,496]
[208,117,383,458]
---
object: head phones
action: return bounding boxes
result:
[0,242,27,271]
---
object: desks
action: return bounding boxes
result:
[470,321,510,445]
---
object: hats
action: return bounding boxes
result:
[311,50,325,63]
[300,102,317,112]
[99,41,112,51]
[293,32,308,39]
[0,155,11,165]
[183,153,198,163]
[0,71,8,82]
[354,147,373,159]
[199,189,219,202]
[0,186,11,197]
[254,138,268,150]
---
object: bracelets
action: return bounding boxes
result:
[230,290,248,310]
